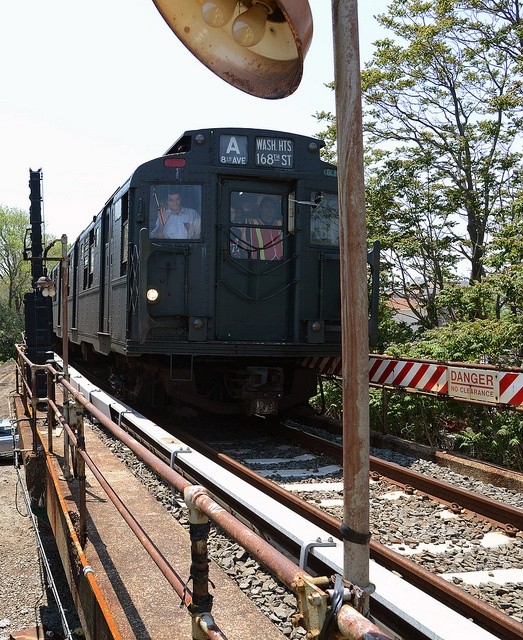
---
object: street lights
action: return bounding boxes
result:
[149,0,369,562]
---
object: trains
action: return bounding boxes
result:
[50,127,380,421]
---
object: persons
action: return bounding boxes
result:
[249,197,284,260]
[230,207,247,258]
[149,189,201,240]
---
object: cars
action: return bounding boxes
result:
[0,422,25,461]
